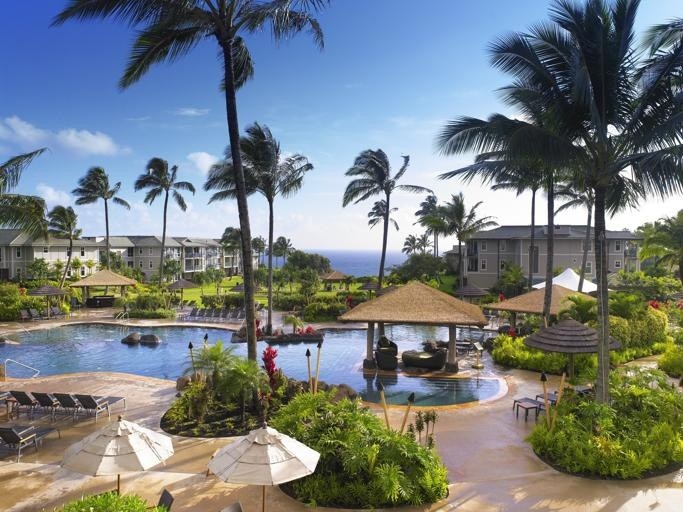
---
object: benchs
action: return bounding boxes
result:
[456,342,482,357]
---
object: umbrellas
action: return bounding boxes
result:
[359,280,377,300]
[532,267,612,294]
[168,277,198,305]
[60,414,175,496]
[28,284,73,317]
[206,422,322,512]
[522,316,622,385]
[231,282,262,304]
[456,282,488,304]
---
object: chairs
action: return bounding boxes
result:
[0,391,126,463]
[219,500,243,512]
[18,307,71,324]
[179,299,265,324]
[375,336,447,371]
[146,488,174,512]
[513,387,592,424]
